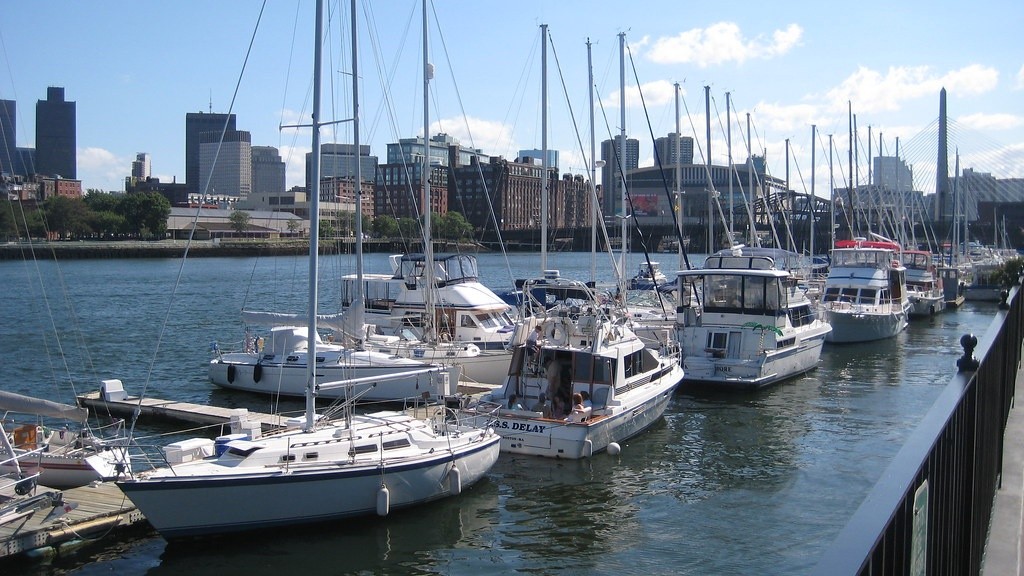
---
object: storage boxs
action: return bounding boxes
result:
[215,434,248,456]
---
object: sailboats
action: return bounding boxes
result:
[1,2,1022,540]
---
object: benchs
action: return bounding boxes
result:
[573,407,593,422]
[478,406,544,418]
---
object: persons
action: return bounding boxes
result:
[640,266,651,278]
[508,329,594,423]
